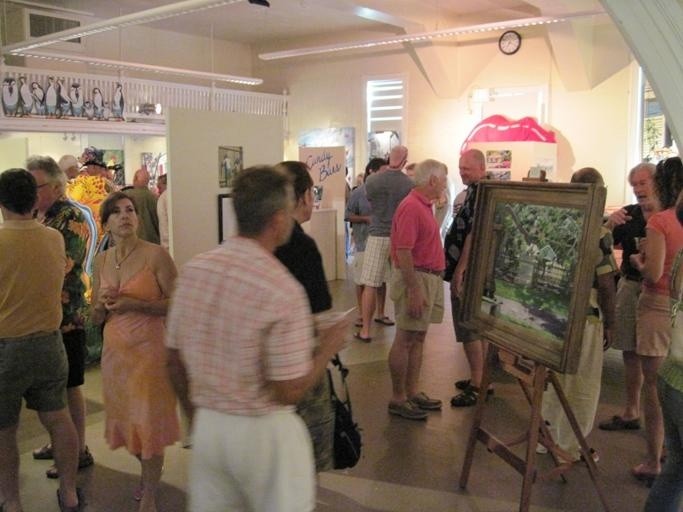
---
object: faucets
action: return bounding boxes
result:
[55,485,86,512]
[449,389,480,408]
[453,379,495,395]
[44,444,95,480]
[31,441,54,459]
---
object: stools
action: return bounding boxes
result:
[415,265,442,277]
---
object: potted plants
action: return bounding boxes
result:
[459,178,607,375]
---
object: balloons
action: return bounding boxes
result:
[499,31,521,54]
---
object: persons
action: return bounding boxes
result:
[157,173,169,252]
[389,160,449,420]
[599,163,668,462]
[0,168,85,512]
[406,163,418,178]
[59,156,80,179]
[165,165,349,512]
[344,158,394,327]
[353,145,416,344]
[630,157,683,487]
[122,169,160,245]
[90,191,179,512]
[345,167,351,263]
[536,168,617,463]
[644,189,683,512]
[272,160,335,473]
[443,149,494,406]
[453,189,467,219]
[26,156,94,479]
[351,172,364,191]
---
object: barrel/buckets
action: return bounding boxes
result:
[374,316,395,325]
[352,330,372,343]
[354,318,363,327]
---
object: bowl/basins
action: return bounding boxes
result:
[112,239,142,269]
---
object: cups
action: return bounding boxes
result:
[37,182,50,188]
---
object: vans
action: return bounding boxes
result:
[629,462,661,489]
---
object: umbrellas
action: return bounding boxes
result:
[387,398,429,421]
[599,414,642,431]
[413,391,442,410]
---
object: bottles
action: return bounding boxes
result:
[388,146,408,169]
